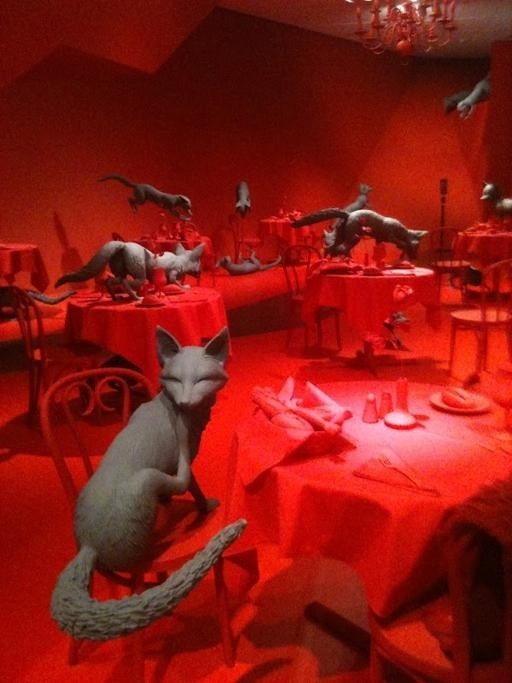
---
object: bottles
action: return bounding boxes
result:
[379,392,393,419]
[362,394,378,423]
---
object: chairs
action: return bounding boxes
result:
[0,217,512,683]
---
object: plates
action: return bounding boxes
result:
[429,387,491,414]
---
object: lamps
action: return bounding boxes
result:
[354,0,458,67]
